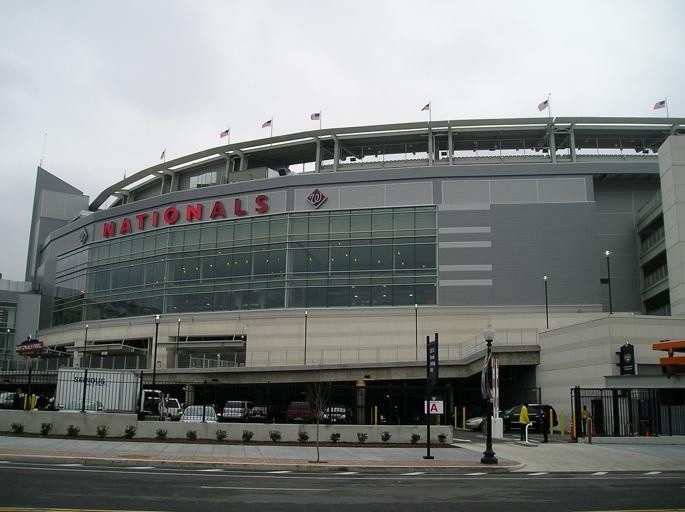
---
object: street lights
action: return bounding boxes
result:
[604,250,613,311]
[543,274,549,329]
[152,314,160,416]
[304,311,307,365]
[83,324,89,365]
[481,319,497,464]
[414,304,418,361]
[175,318,181,368]
[5,329,10,360]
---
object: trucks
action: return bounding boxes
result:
[54,366,164,422]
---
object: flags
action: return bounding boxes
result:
[262,119,271,128]
[538,97,550,111]
[220,129,228,138]
[311,113,320,120]
[421,105,430,113]
[653,98,666,111]
[160,152,164,160]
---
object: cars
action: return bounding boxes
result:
[0,389,53,409]
[287,401,317,424]
[178,406,221,423]
[320,406,354,423]
[247,405,287,423]
[465,411,504,430]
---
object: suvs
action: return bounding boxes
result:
[502,404,560,432]
[222,401,253,422]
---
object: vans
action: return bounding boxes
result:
[158,398,183,421]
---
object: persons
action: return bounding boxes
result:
[537,408,548,443]
[581,404,591,435]
[9,385,56,410]
[519,401,530,441]
